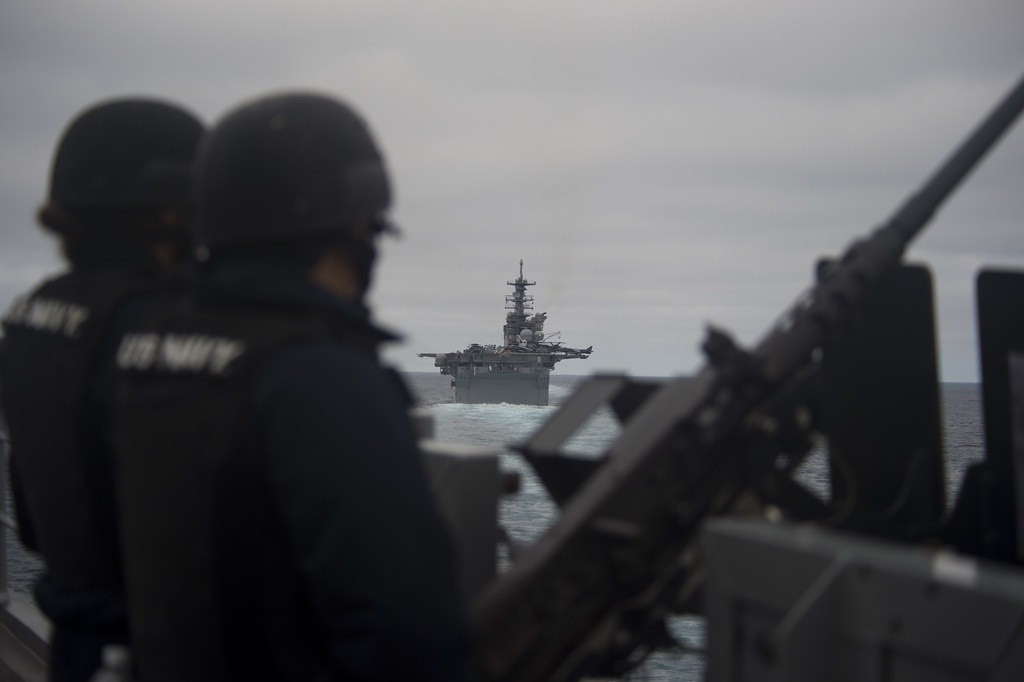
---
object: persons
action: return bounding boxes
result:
[0,93,479,682]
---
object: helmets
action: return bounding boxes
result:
[37,98,208,226]
[182,93,399,241]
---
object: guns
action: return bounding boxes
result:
[470,74,1023,678]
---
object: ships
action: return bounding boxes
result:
[415,258,594,407]
